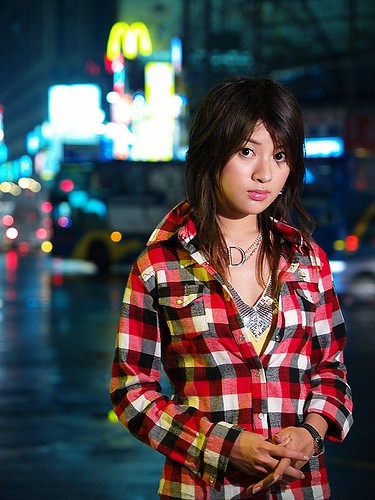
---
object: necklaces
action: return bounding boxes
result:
[229,229,262,267]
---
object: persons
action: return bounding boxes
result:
[110,76,354,500]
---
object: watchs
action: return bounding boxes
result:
[301,423,325,456]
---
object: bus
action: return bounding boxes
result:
[50,157,374,274]
